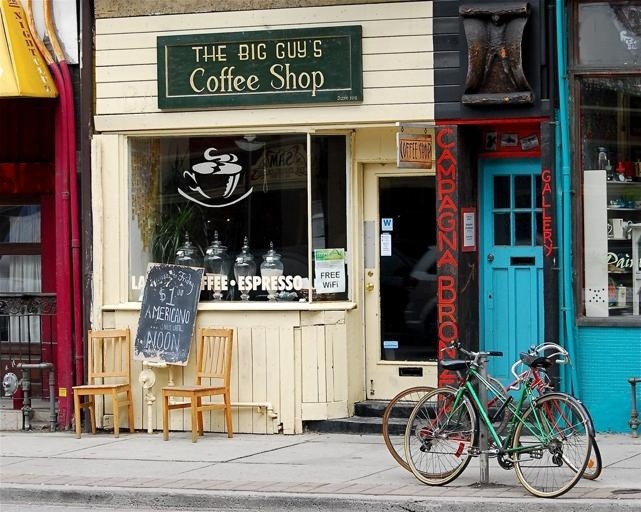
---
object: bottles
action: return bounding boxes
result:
[176,230,284,302]
[616,284,627,307]
[595,146,625,182]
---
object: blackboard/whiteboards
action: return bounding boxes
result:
[132,262,205,367]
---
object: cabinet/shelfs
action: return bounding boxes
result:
[585,169,641,317]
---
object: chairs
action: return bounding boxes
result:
[162,328,234,443]
[71,327,135,439]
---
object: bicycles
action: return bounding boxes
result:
[404,337,597,499]
[382,339,603,482]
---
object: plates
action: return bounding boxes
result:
[607,204,625,209]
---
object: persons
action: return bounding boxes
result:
[472,13,523,93]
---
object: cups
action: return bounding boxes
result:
[607,219,633,240]
